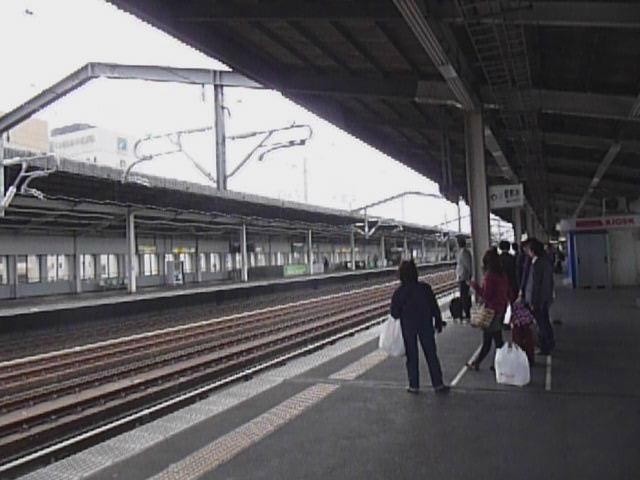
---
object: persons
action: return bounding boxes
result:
[499,238,563,355]
[455,236,473,319]
[390,260,449,393]
[464,251,511,370]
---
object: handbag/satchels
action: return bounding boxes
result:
[378,319,406,357]
[470,301,495,328]
[513,304,534,327]
[449,297,468,320]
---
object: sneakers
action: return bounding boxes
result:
[406,386,419,393]
[435,385,449,392]
[466,359,478,370]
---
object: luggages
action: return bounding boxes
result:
[511,325,535,364]
